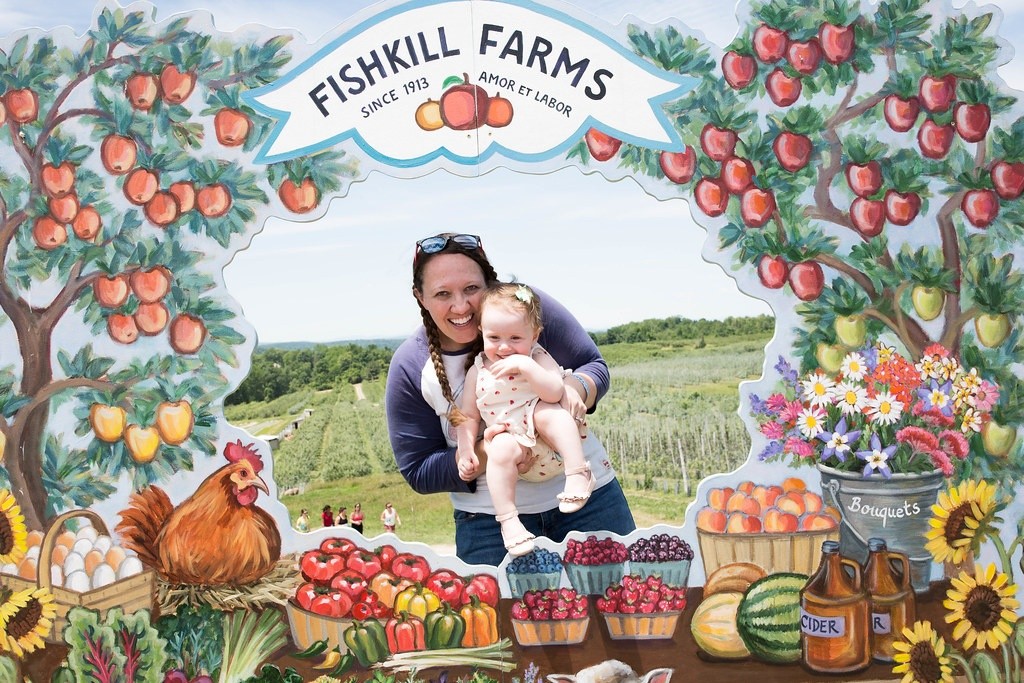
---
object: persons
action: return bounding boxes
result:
[295,509,310,533]
[322,505,335,527]
[456,275,597,556]
[385,230,634,565]
[350,503,365,534]
[380,503,402,534]
[335,507,348,526]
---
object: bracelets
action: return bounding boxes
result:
[569,373,590,405]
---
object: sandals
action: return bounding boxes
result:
[496,510,535,556]
[557,460,596,513]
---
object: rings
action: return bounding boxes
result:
[575,417,584,424]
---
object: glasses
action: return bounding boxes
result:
[415,233,483,263]
[387,506,392,508]
